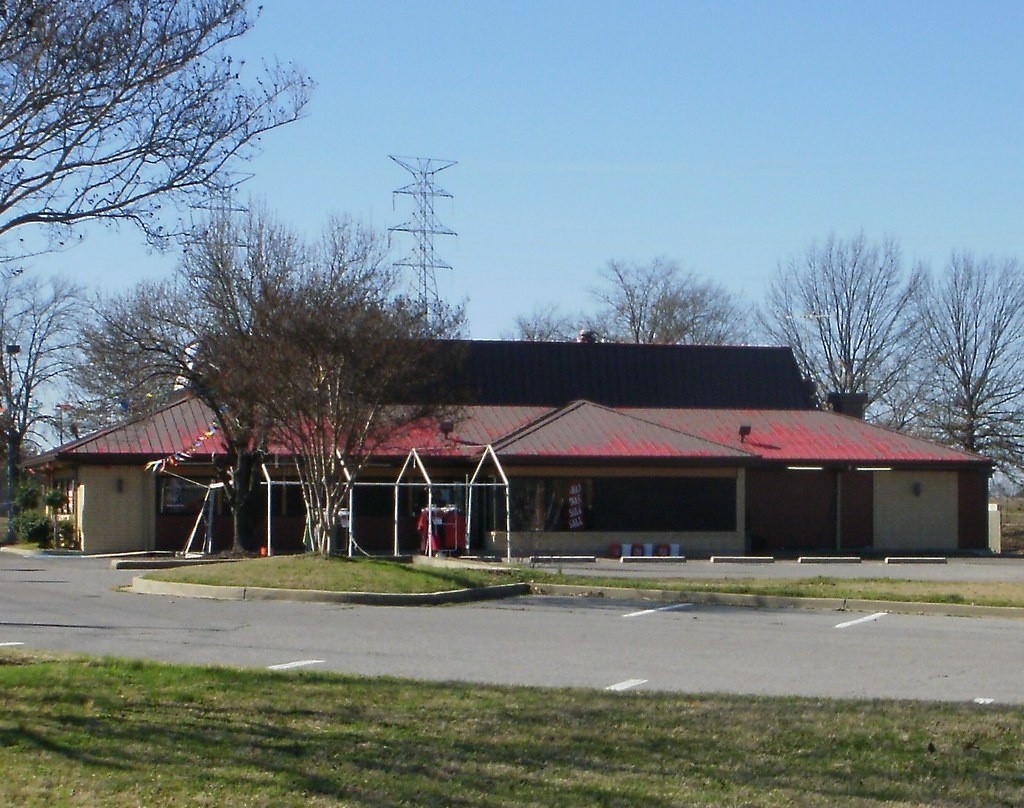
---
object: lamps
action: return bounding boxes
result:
[913,481,921,496]
[116,477,123,492]
[786,466,824,471]
[440,421,454,439]
[855,466,894,471]
[738,424,751,441]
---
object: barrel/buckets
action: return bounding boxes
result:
[260,545,273,557]
[621,543,632,556]
[632,544,642,555]
[610,544,621,558]
[642,544,655,556]
[669,544,680,556]
[656,544,669,556]
